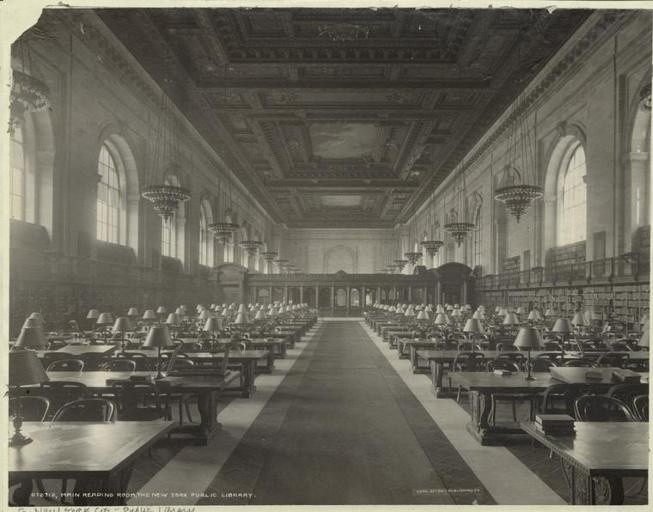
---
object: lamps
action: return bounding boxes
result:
[9,8,288,273]
[386,8,550,275]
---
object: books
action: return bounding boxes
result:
[494,370,512,375]
[131,374,151,381]
[506,283,649,325]
[613,370,642,382]
[534,414,577,437]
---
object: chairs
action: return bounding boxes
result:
[8,298,320,505]
[361,300,647,504]
